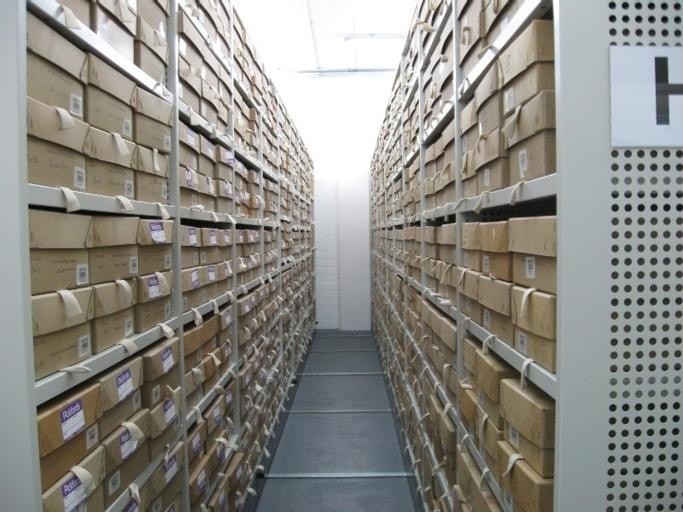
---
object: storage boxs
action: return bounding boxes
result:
[28,0,316,511]
[369,0,556,511]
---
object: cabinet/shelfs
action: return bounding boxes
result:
[369,0,683,511]
[0,0,318,512]
